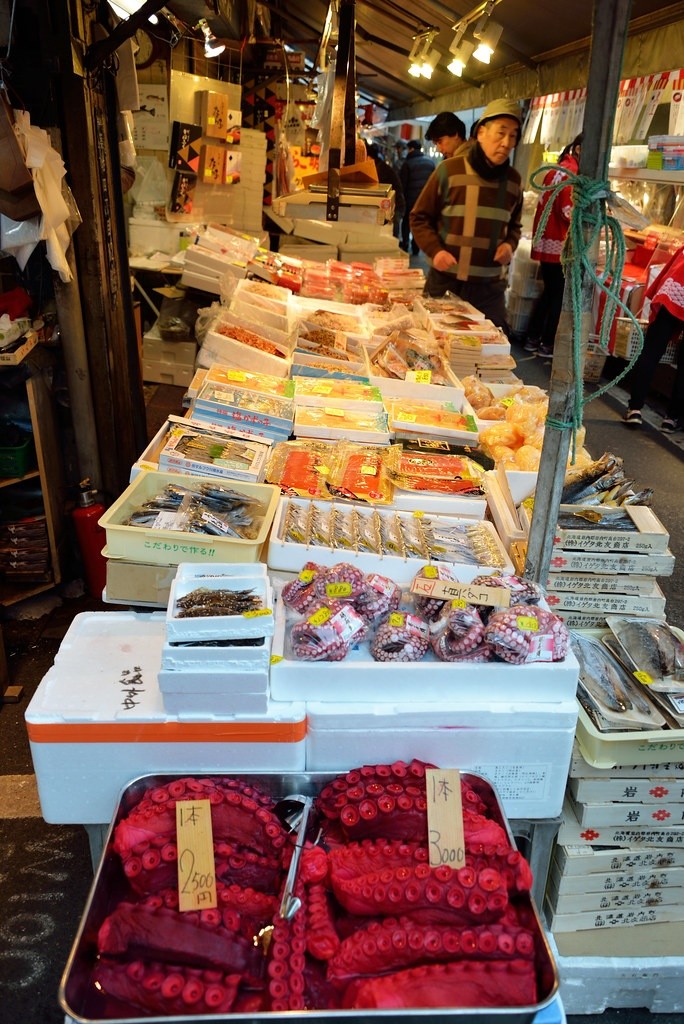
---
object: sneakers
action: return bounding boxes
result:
[621,407,642,425]
[660,415,682,433]
[523,337,541,351]
[536,343,553,358]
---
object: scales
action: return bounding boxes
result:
[271,182,397,225]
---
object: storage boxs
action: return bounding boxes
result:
[24,89,683,1024]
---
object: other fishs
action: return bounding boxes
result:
[569,630,632,712]
[515,451,655,531]
[174,587,262,618]
[122,484,259,539]
[618,618,684,684]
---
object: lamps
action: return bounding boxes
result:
[407,26,441,80]
[194,17,226,59]
[446,0,505,78]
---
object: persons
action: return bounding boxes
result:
[399,140,435,257]
[425,111,480,160]
[363,139,407,238]
[522,133,581,358]
[409,99,523,328]
[620,244,684,433]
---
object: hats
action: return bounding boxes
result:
[392,139,408,148]
[473,98,522,148]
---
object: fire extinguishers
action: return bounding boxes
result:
[66,479,128,611]
